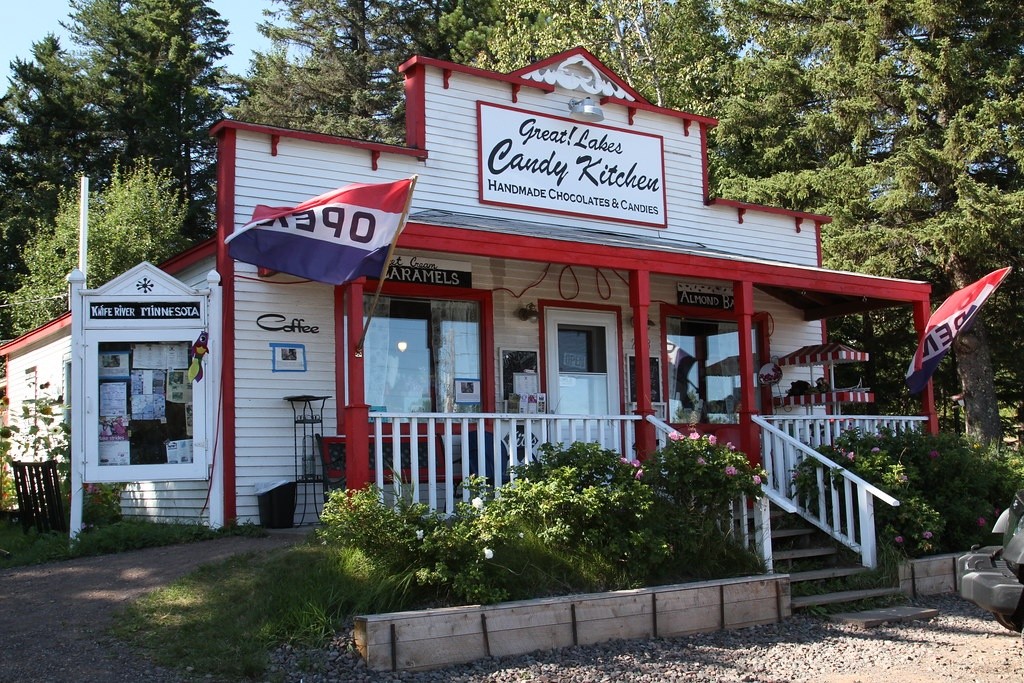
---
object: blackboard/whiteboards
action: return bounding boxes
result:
[625,352,664,404]
[498,347,541,401]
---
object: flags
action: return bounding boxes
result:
[223,175,412,286]
[905,266,1014,388]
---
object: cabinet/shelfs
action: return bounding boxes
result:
[283,394,332,531]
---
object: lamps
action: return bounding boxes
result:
[518,302,539,323]
[568,96,604,123]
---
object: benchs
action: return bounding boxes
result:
[312,433,474,531]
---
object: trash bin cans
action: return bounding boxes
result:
[257,482,296,528]
